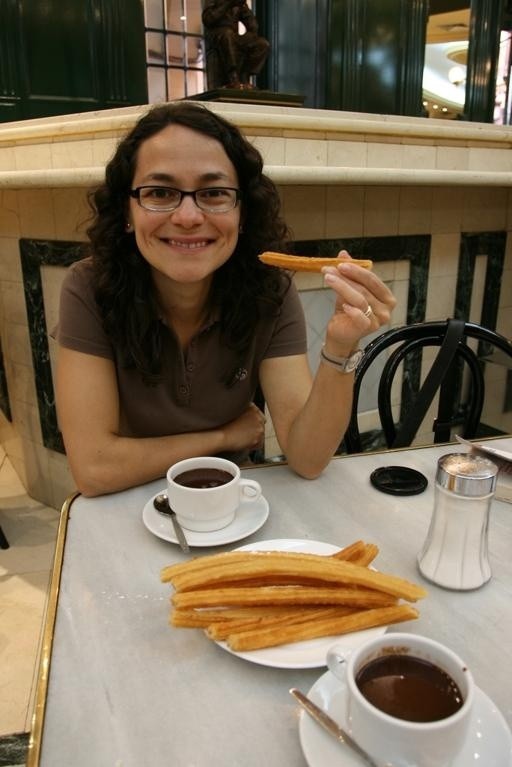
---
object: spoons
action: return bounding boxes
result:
[152,493,189,553]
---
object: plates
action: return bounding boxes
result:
[143,488,269,548]
[300,664,511,767]
[204,540,389,669]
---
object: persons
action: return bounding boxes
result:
[47,100,398,499]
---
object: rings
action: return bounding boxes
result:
[362,304,374,319]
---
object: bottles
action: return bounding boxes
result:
[417,452,499,592]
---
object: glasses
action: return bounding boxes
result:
[130,184,246,214]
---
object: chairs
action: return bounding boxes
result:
[343,319,511,452]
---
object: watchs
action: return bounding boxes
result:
[319,344,365,375]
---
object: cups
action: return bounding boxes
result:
[325,631,476,766]
[167,456,261,533]
